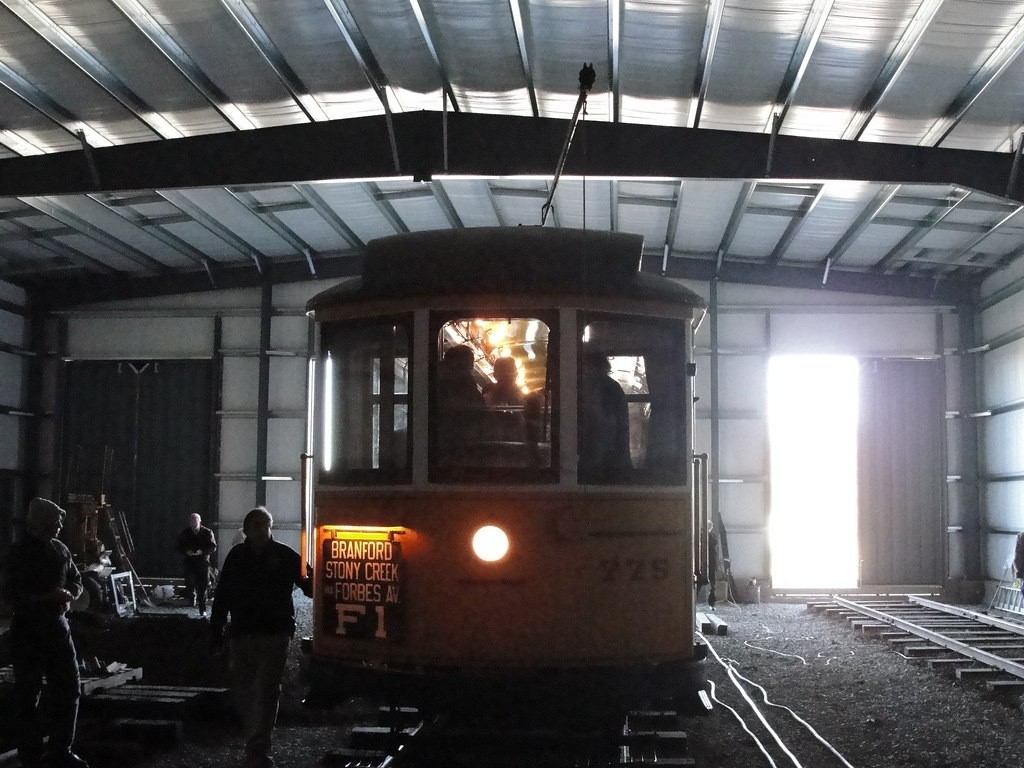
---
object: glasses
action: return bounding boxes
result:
[54,517,64,523]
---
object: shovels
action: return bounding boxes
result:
[118,542,159,608]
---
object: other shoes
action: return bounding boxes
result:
[47,741,90,768]
[246,753,275,768]
[24,759,41,768]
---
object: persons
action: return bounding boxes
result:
[175,512,217,616]
[6,496,89,768]
[207,510,314,768]
[430,339,646,485]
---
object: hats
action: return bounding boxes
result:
[28,497,66,524]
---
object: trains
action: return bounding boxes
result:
[303,225,708,676]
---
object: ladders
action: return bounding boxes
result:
[104,508,130,572]
[992,585,1024,616]
